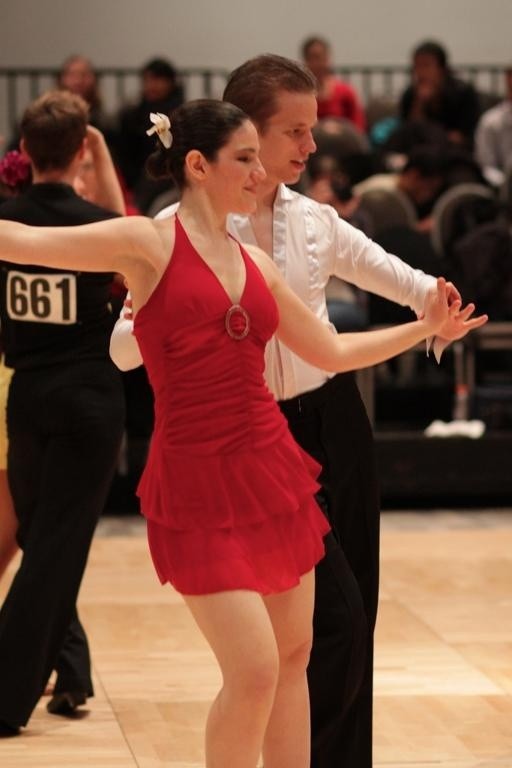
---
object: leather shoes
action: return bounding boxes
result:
[47,688,87,715]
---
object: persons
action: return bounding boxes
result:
[4,33,511,434]
[1,99,130,738]
[3,91,129,696]
[108,52,488,767]
[5,99,462,767]
